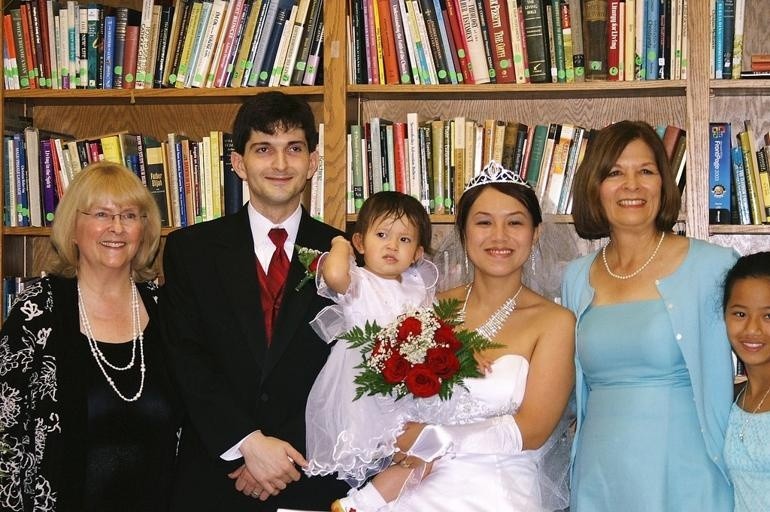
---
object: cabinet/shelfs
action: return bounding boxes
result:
[338,2,695,237]
[0,0,336,239]
[700,2,769,240]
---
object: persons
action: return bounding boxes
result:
[723,251,770,510]
[372,171,575,512]
[559,121,741,511]
[163,90,362,511]
[2,163,185,510]
[321,190,442,510]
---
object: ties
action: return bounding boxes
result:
[267,227,292,314]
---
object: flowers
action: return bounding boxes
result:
[333,298,508,403]
[290,243,326,292]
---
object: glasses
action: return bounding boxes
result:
[75,206,148,225]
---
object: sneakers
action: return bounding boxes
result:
[330,496,364,512]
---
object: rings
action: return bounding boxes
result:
[253,491,259,497]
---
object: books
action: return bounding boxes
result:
[3,270,47,322]
[708,0,769,225]
[347,113,686,216]
[443,251,462,290]
[345,0,687,85]
[3,125,325,227]
[2,0,324,88]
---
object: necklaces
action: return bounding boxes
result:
[737,375,769,441]
[457,281,523,348]
[75,276,153,404]
